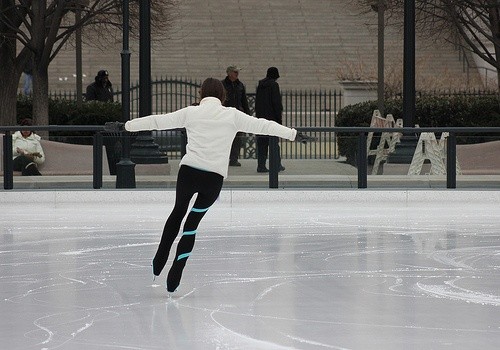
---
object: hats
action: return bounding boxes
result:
[98,70,108,81]
[267,67,280,78]
[21,119,32,125]
[226,65,242,72]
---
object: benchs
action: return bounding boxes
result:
[456,141,500,175]
[0,134,111,176]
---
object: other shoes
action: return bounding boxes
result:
[229,161,241,166]
[280,167,285,171]
[257,167,269,172]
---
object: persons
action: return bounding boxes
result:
[86,70,114,102]
[12,119,45,176]
[255,67,285,173]
[23,72,33,97]
[220,65,251,166]
[104,77,317,298]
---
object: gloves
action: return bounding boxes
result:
[104,122,127,133]
[296,132,318,144]
